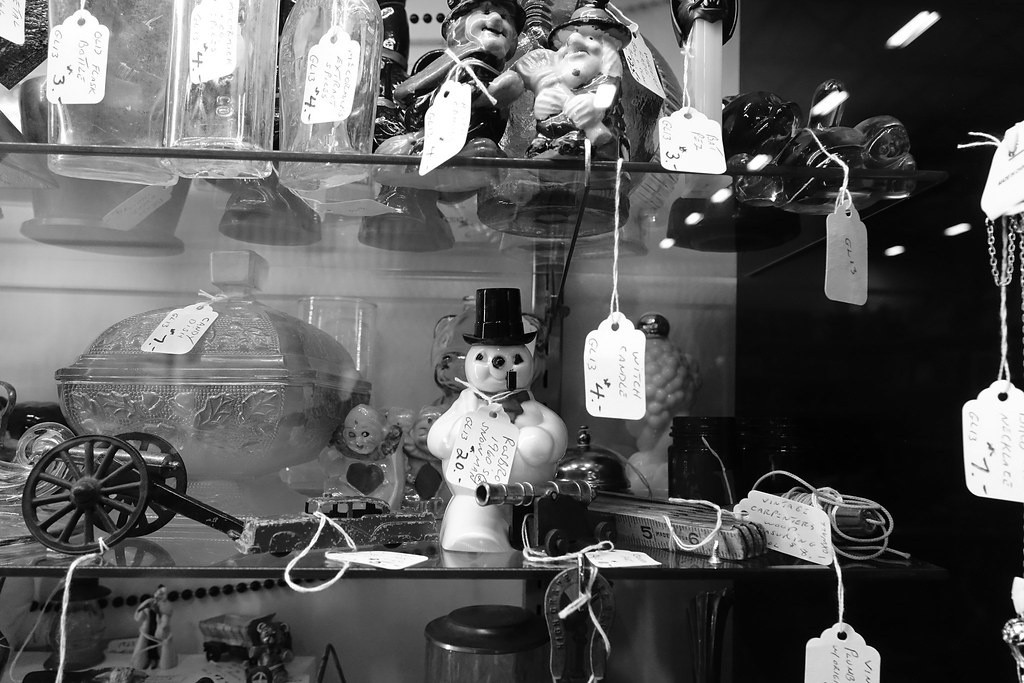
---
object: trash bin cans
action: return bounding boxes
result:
[424,604,550,683]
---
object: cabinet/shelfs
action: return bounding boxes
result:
[0,1,1024,683]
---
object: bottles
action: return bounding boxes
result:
[742,416,806,501]
[666,415,746,514]
[166,1,278,180]
[279,0,383,195]
[45,0,184,186]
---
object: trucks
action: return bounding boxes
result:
[199,613,296,666]
